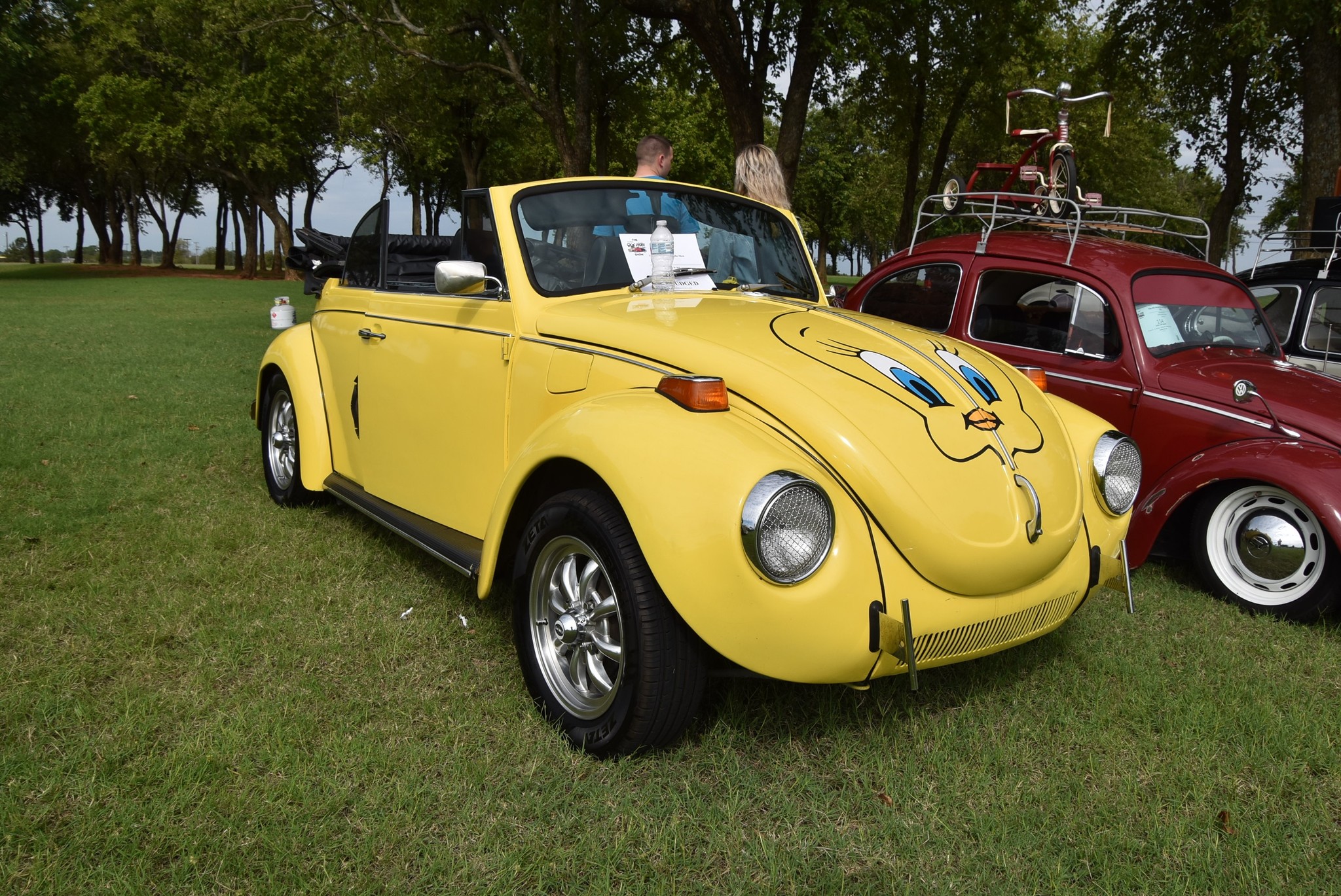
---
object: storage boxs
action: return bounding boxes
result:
[1308,166,1341,254]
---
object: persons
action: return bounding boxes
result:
[592,133,701,237]
[707,143,793,286]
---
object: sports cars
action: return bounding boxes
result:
[246,176,1142,760]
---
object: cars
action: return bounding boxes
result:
[1169,229,1341,383]
[830,190,1341,626]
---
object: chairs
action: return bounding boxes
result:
[524,238,573,291]
[973,304,1039,349]
[1306,317,1341,352]
[1036,309,1106,355]
[447,228,510,299]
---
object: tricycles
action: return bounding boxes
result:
[940,81,1119,224]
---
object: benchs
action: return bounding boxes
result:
[864,283,1062,329]
